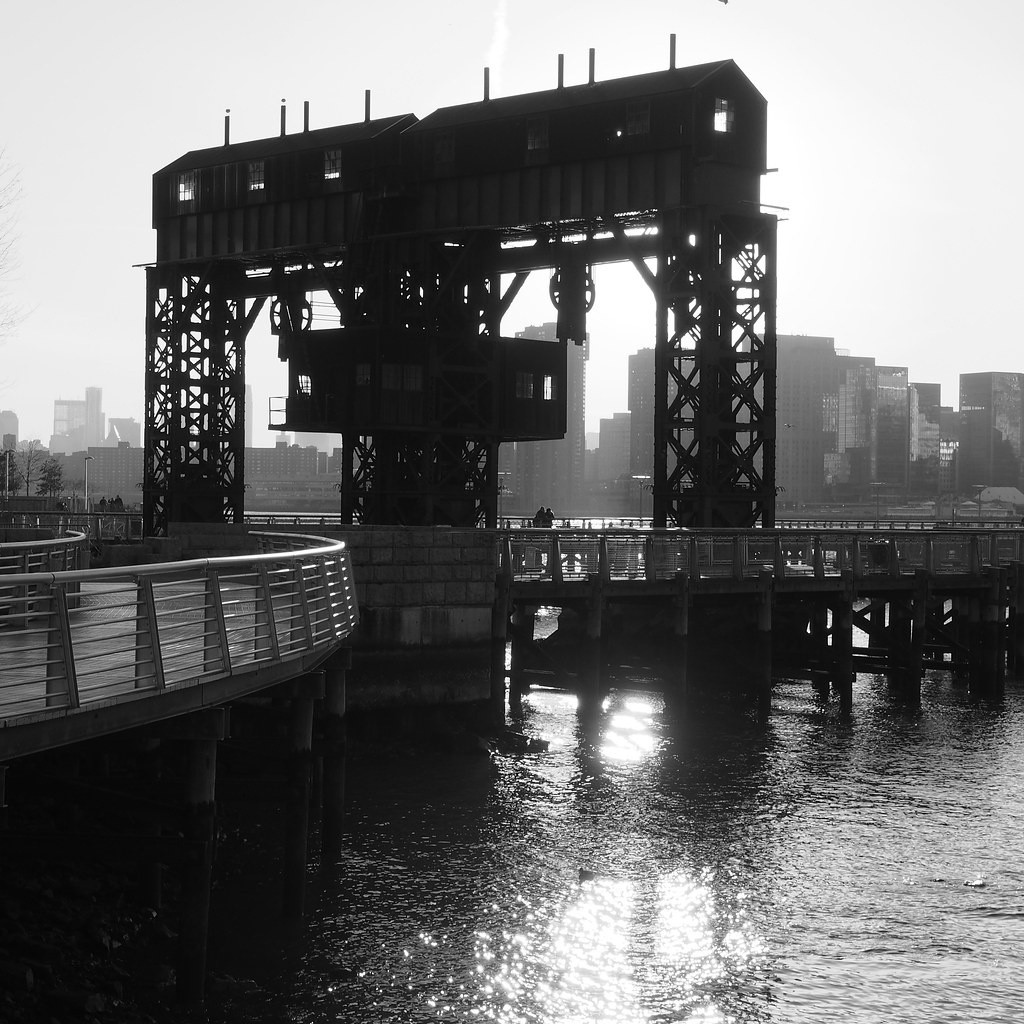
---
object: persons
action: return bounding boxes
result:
[60,503,68,511]
[100,497,107,512]
[533,507,554,528]
[108,495,123,511]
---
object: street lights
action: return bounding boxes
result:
[85,457,94,512]
[632,476,650,527]
[498,472,512,530]
[971,485,989,520]
[869,483,886,528]
[4,449,16,501]
